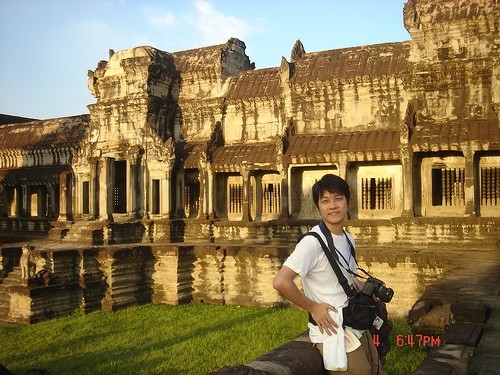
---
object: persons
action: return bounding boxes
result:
[273,174,386,375]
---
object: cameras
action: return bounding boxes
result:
[362,277,394,303]
